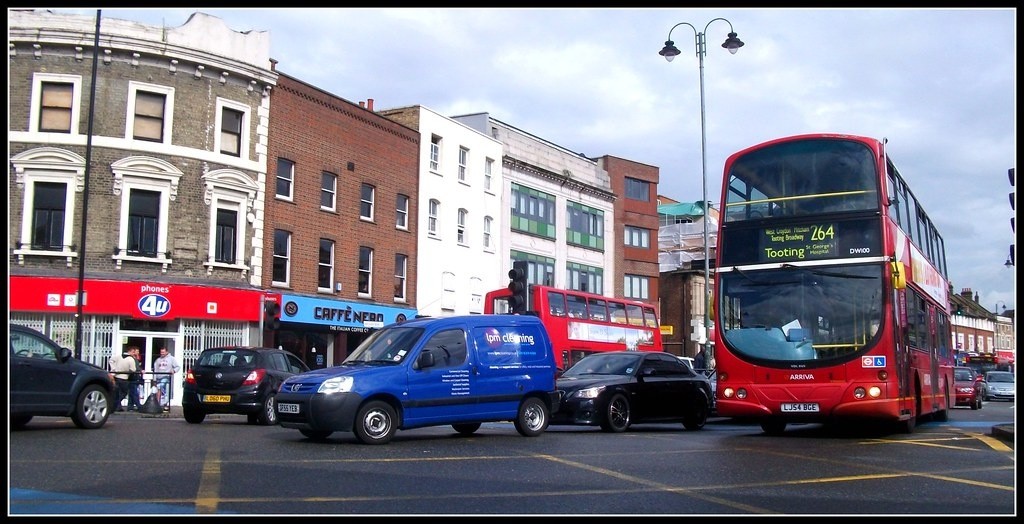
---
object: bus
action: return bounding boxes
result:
[485,287,664,380]
[709,134,953,435]
[966,356,1014,391]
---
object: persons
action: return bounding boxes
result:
[107,346,143,411]
[692,345,705,374]
[153,346,181,407]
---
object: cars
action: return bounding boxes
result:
[550,351,713,431]
[707,368,717,409]
[182,344,311,425]
[644,356,695,374]
[980,371,1015,402]
[10,322,120,431]
[950,367,985,409]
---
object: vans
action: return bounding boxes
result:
[275,312,561,445]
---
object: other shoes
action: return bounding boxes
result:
[115,406,124,411]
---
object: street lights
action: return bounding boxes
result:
[658,17,745,369]
[995,300,1007,349]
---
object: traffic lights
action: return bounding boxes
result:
[957,305,962,315]
[508,259,529,313]
